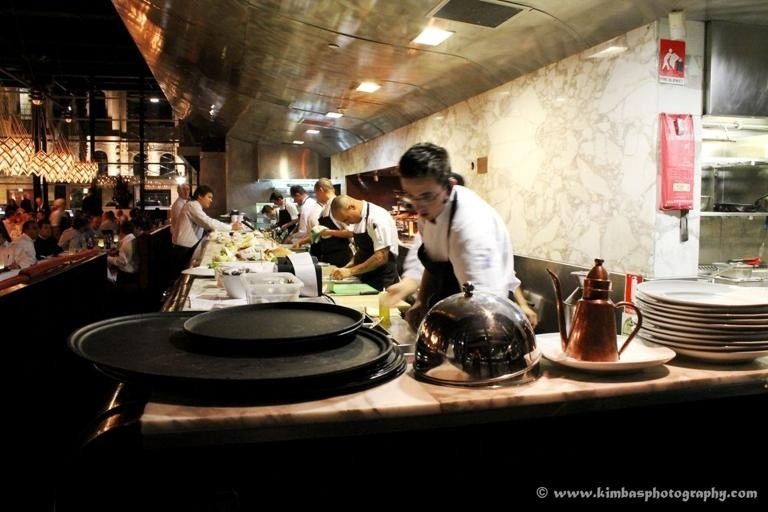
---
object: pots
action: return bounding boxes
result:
[714,194,768,212]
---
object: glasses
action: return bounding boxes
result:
[401,187,446,206]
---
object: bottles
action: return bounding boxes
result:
[378,287,391,325]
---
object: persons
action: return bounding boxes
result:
[1,191,166,284]
[261,144,539,330]
[170,184,241,275]
[168,182,191,244]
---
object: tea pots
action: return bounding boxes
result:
[543,255,643,365]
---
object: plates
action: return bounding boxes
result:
[533,278,768,378]
[177,222,355,284]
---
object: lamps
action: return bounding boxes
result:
[0,70,98,183]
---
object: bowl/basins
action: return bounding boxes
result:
[223,276,248,298]
[700,195,712,211]
